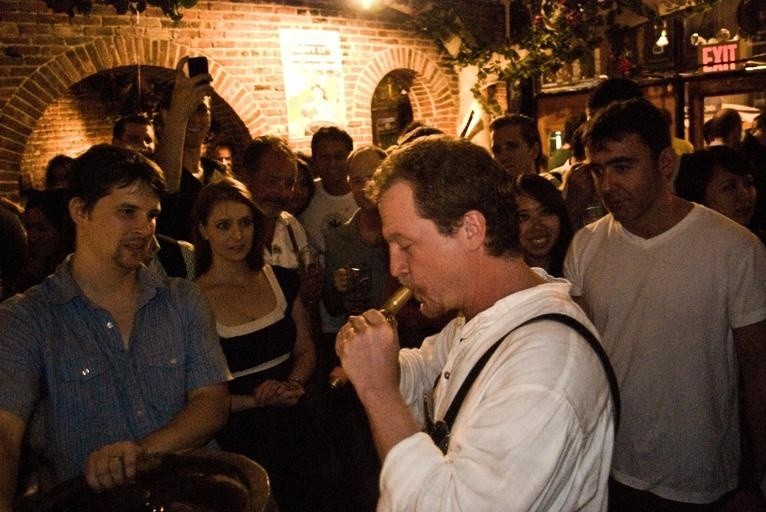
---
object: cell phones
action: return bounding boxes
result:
[187,56,210,86]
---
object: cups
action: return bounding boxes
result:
[348,265,372,306]
[296,247,319,275]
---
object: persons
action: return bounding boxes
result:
[0,52,766,511]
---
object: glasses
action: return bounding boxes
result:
[196,104,212,114]
